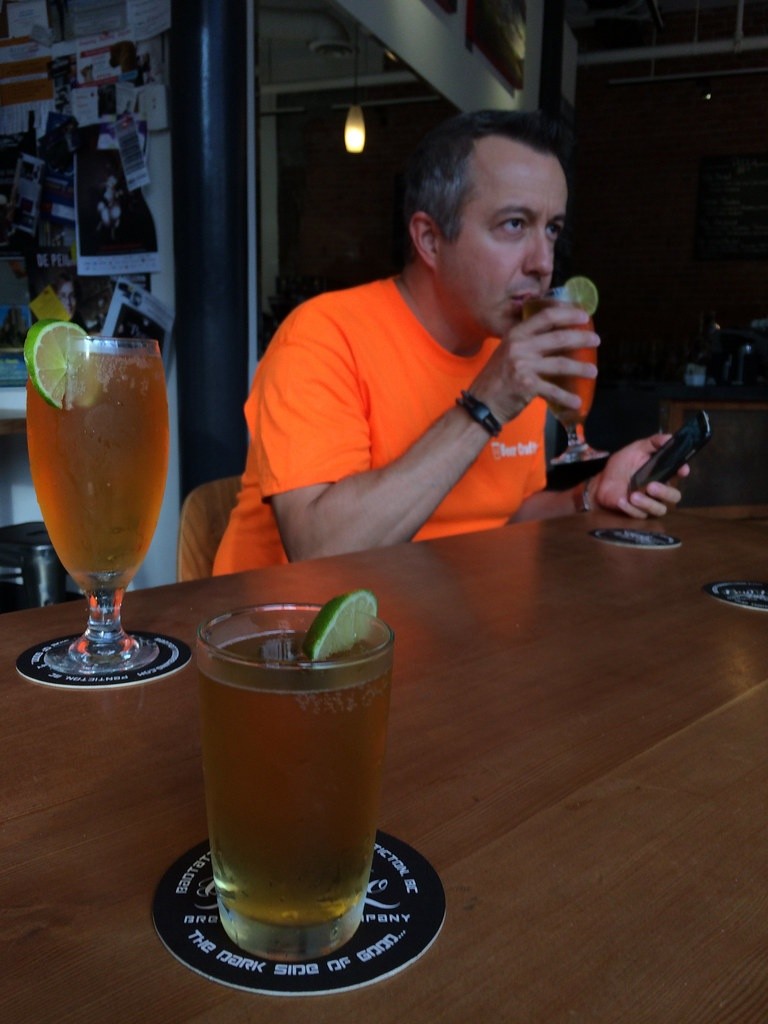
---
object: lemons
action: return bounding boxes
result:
[303,587,379,660]
[564,276,598,317]
[23,318,98,409]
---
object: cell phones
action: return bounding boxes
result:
[628,409,712,499]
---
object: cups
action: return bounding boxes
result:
[194,602,396,963]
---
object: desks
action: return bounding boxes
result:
[0,499,768,1024]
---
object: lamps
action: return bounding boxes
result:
[344,20,366,154]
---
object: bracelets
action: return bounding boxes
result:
[453,388,505,439]
[572,474,597,514]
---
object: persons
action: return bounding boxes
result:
[47,270,112,335]
[211,109,691,577]
[109,40,144,87]
[596,307,725,389]
[101,172,157,254]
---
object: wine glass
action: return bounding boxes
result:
[521,285,609,465]
[26,336,172,677]
